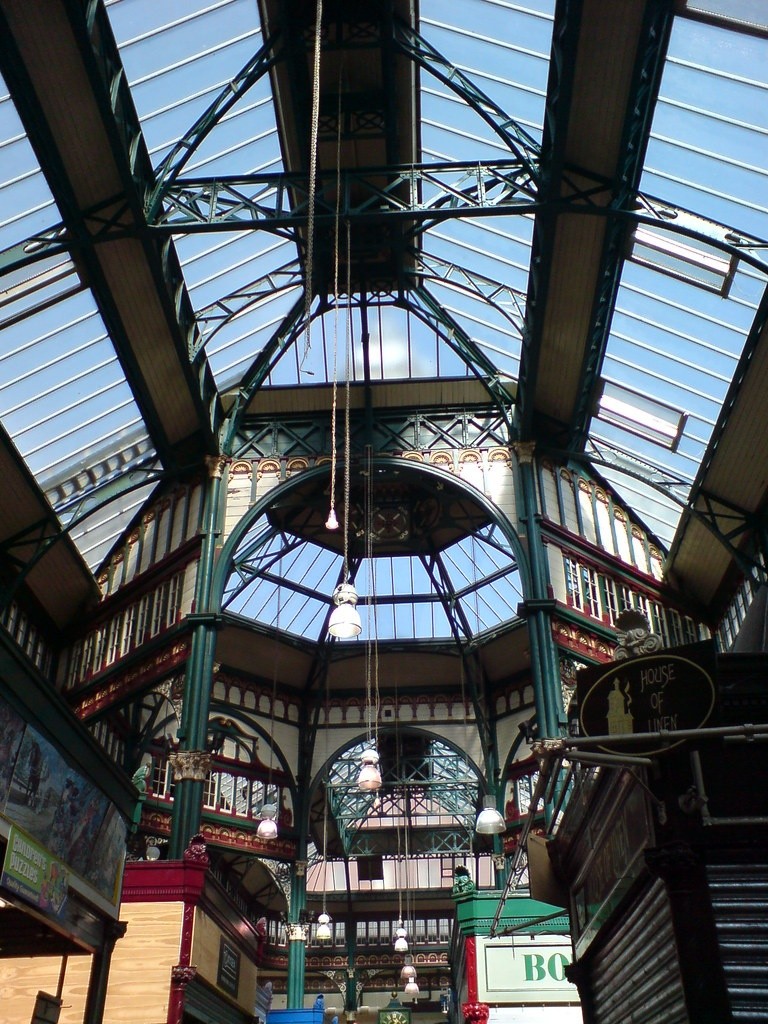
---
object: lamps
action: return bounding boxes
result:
[469,515,508,837]
[257,505,279,840]
[400,759,419,980]
[392,674,410,953]
[328,222,364,636]
[356,446,383,796]
[405,858,419,995]
[315,666,333,940]
